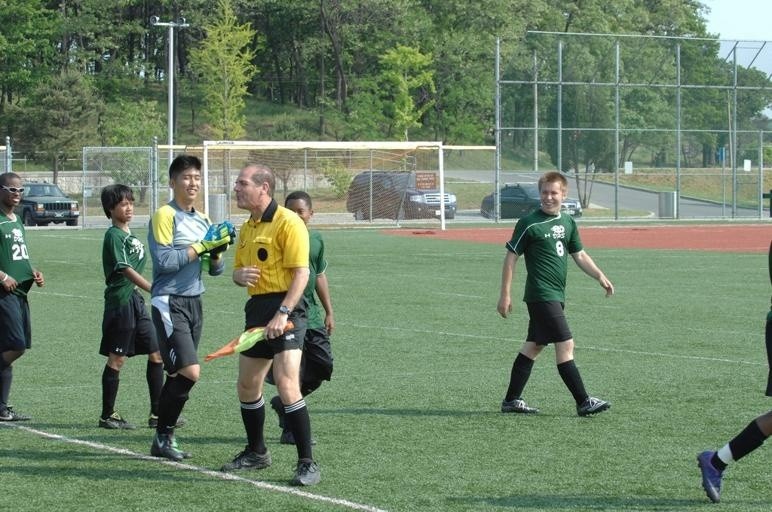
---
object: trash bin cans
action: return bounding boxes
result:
[659,191,677,219]
[209,194,227,224]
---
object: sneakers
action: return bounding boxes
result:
[1,407,32,421]
[98,411,136,430]
[576,396,611,416]
[288,459,321,486]
[270,395,316,446]
[148,433,196,461]
[148,412,188,428]
[697,450,722,504]
[219,444,272,473]
[501,398,541,413]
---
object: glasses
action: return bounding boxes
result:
[1,183,25,196]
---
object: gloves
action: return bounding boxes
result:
[189,220,237,261]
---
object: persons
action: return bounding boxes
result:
[0,173,34,421]
[266,189,334,446]
[496,170,614,417]
[98,185,188,428]
[220,165,320,487]
[146,155,237,460]
[696,189,772,505]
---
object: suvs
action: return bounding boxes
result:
[345,166,461,226]
[11,181,80,227]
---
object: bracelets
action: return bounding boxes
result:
[0,272,9,283]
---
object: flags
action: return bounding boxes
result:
[205,320,295,361]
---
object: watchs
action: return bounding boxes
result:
[278,304,292,317]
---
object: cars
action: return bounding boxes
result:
[481,180,585,220]
